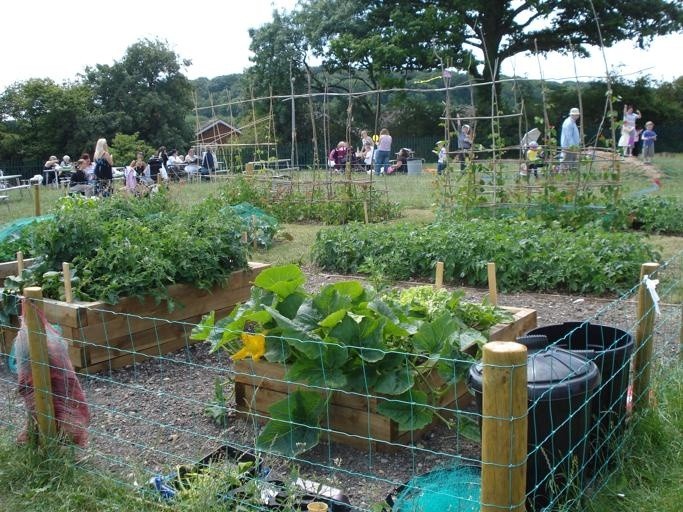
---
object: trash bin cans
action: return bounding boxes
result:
[406,158,422,175]
[468,321,633,512]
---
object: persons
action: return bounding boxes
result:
[617,104,644,157]
[558,107,580,171]
[519,120,558,178]
[359,130,376,174]
[455,112,474,175]
[360,144,372,175]
[373,127,392,177]
[639,120,658,165]
[390,147,409,177]
[431,140,448,175]
[327,141,351,174]
[38,137,218,200]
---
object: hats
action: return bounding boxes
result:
[568,108,580,115]
[529,142,538,147]
[644,120,654,127]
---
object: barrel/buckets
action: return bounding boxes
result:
[407,159,422,177]
[523,320,635,472]
[467,339,598,506]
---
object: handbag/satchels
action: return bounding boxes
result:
[93,152,110,179]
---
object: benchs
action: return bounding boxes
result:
[0,167,126,203]
[242,158,299,181]
[166,162,228,184]
